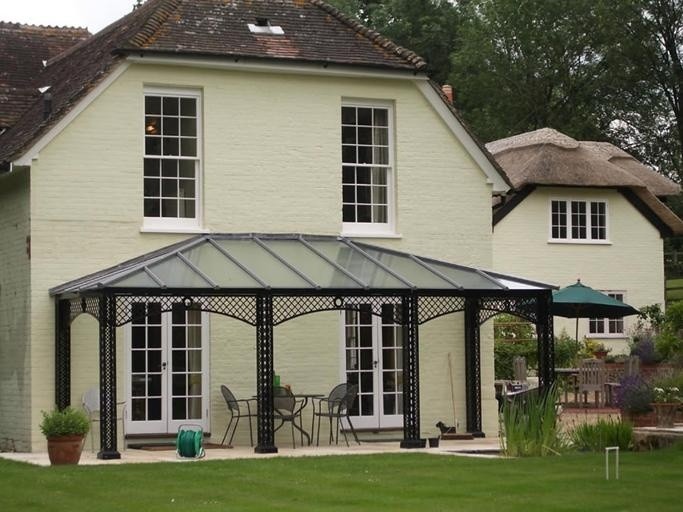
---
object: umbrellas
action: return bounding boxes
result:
[514,277,642,364]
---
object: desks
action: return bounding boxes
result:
[554,366,602,405]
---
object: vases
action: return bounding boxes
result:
[648,403,682,428]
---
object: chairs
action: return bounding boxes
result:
[219,382,360,449]
[81,388,127,454]
[571,358,608,409]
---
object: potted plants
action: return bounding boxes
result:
[38,403,90,466]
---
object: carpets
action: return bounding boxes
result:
[129,442,233,451]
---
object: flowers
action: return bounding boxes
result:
[652,385,683,404]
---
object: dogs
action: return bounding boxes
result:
[435,421,459,434]
